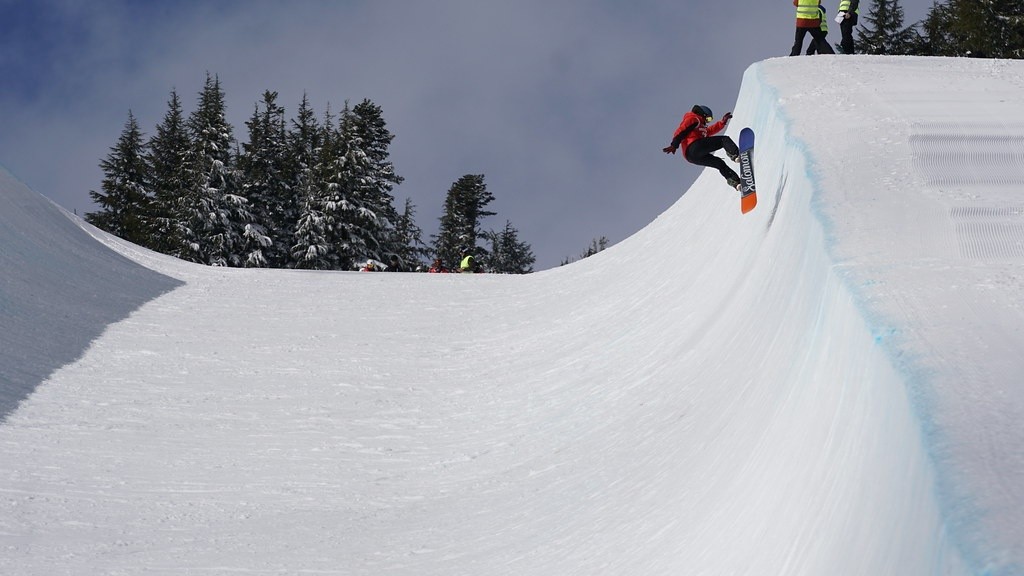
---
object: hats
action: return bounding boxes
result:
[367,259,373,265]
[700,106,713,116]
[460,246,469,253]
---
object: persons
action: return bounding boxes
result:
[364,251,476,273]
[789,0,860,56]
[662,105,742,191]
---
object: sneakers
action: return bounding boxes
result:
[736,179,741,191]
[734,155,740,163]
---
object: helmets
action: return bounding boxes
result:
[436,259,442,263]
[416,266,423,270]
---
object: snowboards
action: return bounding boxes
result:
[738,128,757,214]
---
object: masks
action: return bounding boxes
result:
[706,117,712,123]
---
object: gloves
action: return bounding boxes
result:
[663,146,676,154]
[722,111,732,125]
